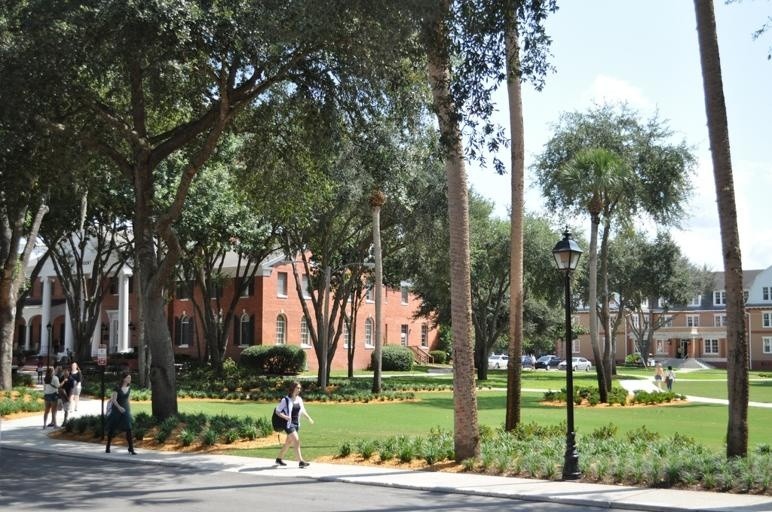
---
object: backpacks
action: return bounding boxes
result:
[271,397,289,432]
[106,397,114,416]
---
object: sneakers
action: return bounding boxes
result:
[275,458,286,466]
[299,461,310,468]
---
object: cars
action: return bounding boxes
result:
[484,347,595,376]
[13,363,125,394]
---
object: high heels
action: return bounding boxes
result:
[127,449,137,456]
[106,445,110,453]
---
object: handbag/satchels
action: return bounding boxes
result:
[58,388,69,403]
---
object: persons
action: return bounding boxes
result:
[107,372,138,455]
[666,366,675,390]
[655,363,665,389]
[275,381,314,467]
[37,348,82,430]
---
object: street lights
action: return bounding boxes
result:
[46,318,55,384]
[549,227,589,480]
